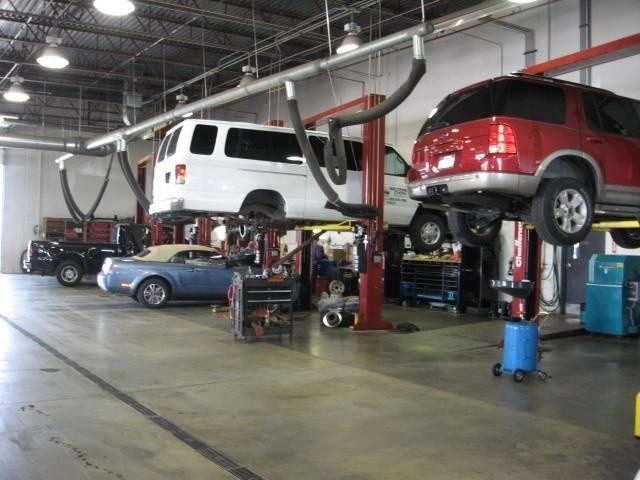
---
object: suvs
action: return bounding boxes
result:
[405,69,640,249]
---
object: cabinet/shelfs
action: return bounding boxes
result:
[398,259,475,311]
[43,217,112,241]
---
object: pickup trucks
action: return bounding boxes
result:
[22,221,191,288]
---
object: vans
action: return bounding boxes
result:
[146,117,458,255]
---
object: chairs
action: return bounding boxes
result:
[172,256,216,266]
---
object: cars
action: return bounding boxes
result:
[96,243,294,310]
